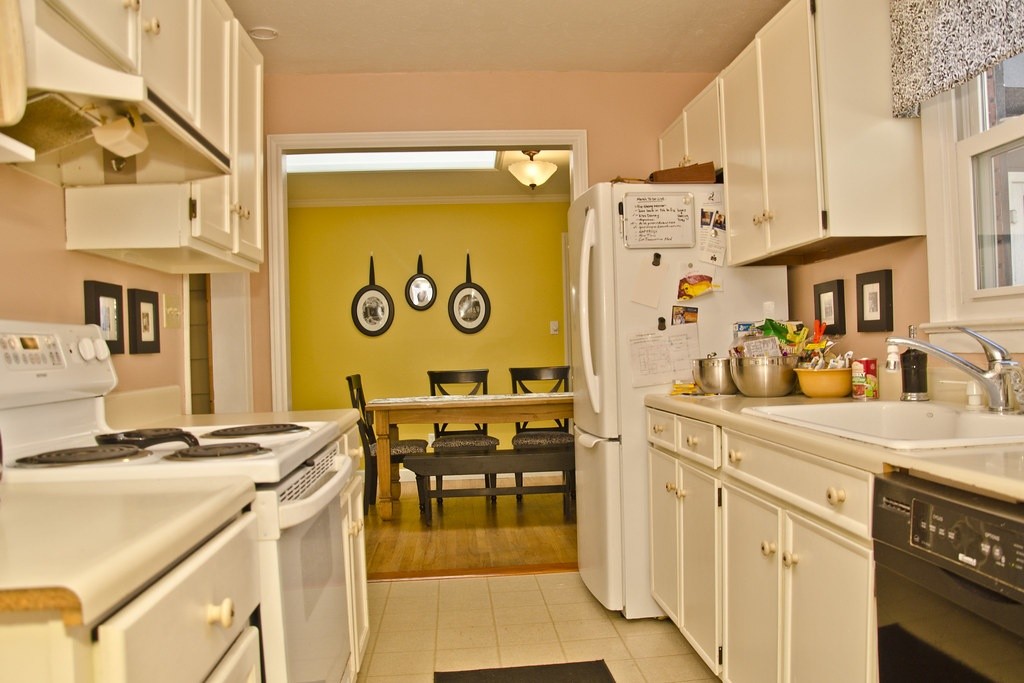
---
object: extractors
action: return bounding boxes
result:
[0,76,233,192]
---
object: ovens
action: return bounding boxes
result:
[250,448,359,682]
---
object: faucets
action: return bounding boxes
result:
[883,326,1017,413]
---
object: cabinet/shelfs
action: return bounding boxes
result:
[1,0,264,274]
[89,425,370,683]
[644,407,883,683]
[658,0,928,271]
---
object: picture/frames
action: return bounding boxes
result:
[83,280,124,354]
[857,269,894,334]
[813,278,846,335]
[126,288,160,354]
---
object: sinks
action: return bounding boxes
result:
[741,401,1023,449]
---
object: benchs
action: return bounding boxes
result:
[403,446,577,528]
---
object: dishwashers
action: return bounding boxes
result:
[874,471,1024,683]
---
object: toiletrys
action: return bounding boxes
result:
[879,335,903,401]
[900,324,929,400]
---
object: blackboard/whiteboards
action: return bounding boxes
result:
[623,191,696,250]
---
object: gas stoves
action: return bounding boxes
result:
[0,320,361,491]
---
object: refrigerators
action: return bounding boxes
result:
[567,181,790,625]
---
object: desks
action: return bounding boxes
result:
[365,392,574,521]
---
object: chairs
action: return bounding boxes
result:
[346,366,571,514]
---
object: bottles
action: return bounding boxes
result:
[900,327,931,404]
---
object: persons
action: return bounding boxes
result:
[367,300,382,323]
[675,311,686,324]
[702,213,722,226]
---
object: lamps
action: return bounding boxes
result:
[507,150,558,191]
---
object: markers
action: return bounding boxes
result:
[619,202,622,237]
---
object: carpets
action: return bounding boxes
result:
[433,659,618,683]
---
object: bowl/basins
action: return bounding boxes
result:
[727,356,800,396]
[691,356,741,396]
[792,367,855,398]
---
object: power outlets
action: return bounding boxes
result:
[429,434,436,447]
